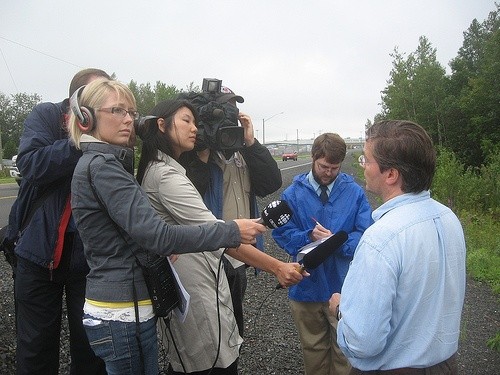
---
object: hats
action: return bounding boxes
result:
[214,86,244,103]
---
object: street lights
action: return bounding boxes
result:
[262,111,284,145]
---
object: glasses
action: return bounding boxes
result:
[358,154,376,168]
[99,106,140,120]
[316,161,341,172]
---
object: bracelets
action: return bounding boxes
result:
[336,304,341,321]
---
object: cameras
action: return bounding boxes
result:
[134,116,161,143]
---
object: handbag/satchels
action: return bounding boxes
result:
[0,224,19,267]
[143,256,180,317]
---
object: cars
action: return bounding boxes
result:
[282,148,298,161]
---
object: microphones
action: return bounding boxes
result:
[255,199,293,230]
[276,231,349,290]
[212,109,224,118]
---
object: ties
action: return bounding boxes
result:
[319,186,328,206]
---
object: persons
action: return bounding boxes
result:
[178,85,283,353]
[72,80,268,375]
[272,133,375,375]
[328,119,467,375]
[0,69,115,375]
[137,100,311,375]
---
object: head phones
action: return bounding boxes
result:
[69,85,140,133]
